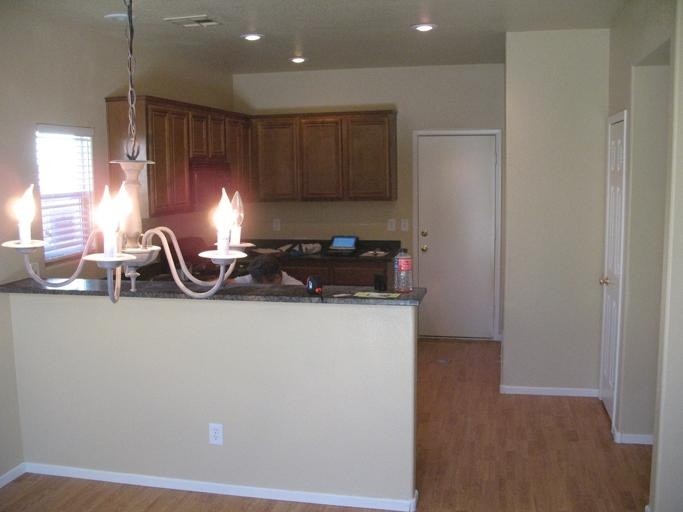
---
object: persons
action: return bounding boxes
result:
[214,255,305,286]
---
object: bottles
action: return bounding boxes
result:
[393,248,412,292]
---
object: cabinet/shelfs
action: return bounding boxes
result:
[104,106,191,216]
[341,116,398,201]
[189,108,226,160]
[249,116,342,201]
[224,118,247,204]
[286,261,389,292]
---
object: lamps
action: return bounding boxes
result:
[0,1,256,305]
